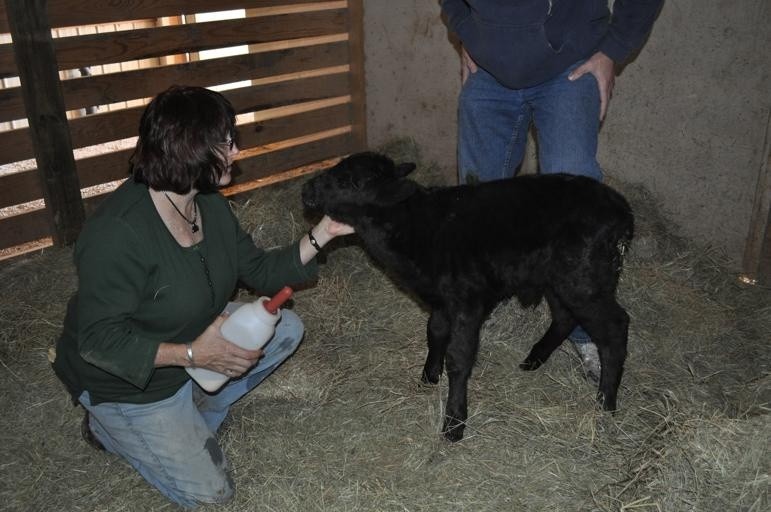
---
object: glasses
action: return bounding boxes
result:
[216,137,233,151]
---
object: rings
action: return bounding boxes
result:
[227,370,233,378]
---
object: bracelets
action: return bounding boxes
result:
[308,228,322,253]
[185,340,196,370]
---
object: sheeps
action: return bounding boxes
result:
[300,150,635,443]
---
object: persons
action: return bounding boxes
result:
[436,1,667,387]
[49,84,305,507]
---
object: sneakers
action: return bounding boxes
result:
[80,411,105,452]
[574,338,601,382]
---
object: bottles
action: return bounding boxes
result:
[180,285,295,396]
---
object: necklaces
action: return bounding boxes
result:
[162,190,200,234]
